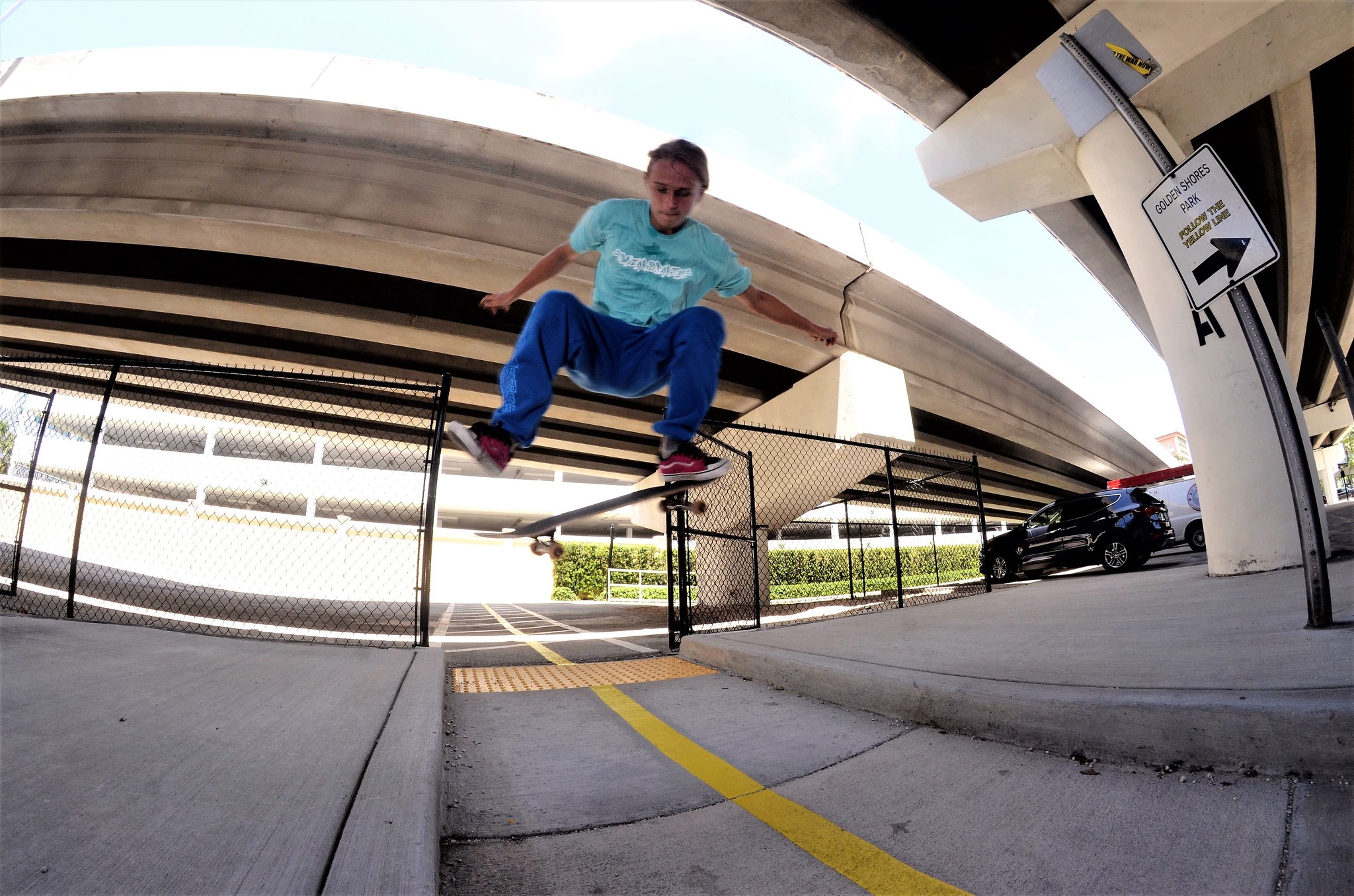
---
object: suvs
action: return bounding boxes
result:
[976,487,1176,584]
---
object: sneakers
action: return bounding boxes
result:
[446,420,515,478]
[657,442,730,481]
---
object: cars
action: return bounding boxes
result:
[1322,484,1354,504]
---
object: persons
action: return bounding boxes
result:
[449,139,839,483]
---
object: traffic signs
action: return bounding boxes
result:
[1140,142,1281,312]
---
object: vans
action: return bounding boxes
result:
[1146,476,1206,553]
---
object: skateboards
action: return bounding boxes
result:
[474,474,721,560]
